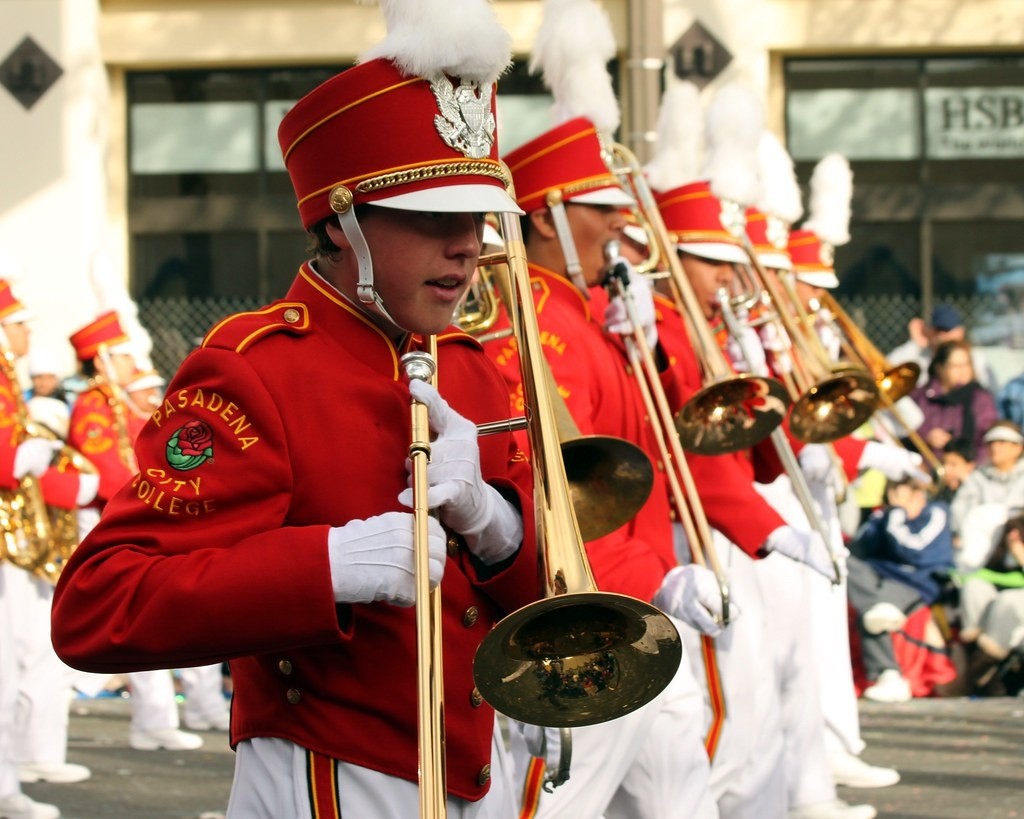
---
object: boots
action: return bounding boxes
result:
[927,626,1005,699]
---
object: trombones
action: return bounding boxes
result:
[714,241,885,591]
[809,290,949,495]
[394,154,690,817]
[604,139,797,627]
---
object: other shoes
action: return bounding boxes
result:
[809,798,876,819]
[862,603,906,634]
[129,726,203,750]
[19,761,90,783]
[0,792,60,819]
[863,670,911,704]
[836,751,900,787]
[185,697,229,732]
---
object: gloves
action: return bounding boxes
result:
[798,442,831,484]
[766,523,852,583]
[396,377,526,566]
[329,512,448,609]
[729,325,768,379]
[873,393,923,440]
[819,326,842,358]
[855,441,922,482]
[596,256,660,360]
[654,560,730,640]
[760,316,790,372]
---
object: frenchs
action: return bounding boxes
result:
[2,421,82,588]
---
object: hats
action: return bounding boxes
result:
[930,307,965,331]
[0,278,29,324]
[70,309,141,361]
[610,37,863,287]
[501,0,637,213]
[275,0,525,234]
[983,426,1022,443]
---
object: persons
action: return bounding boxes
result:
[52,1,546,819]
[845,307,1024,702]
[461,0,738,819]
[621,83,901,819]
[0,278,231,819]
